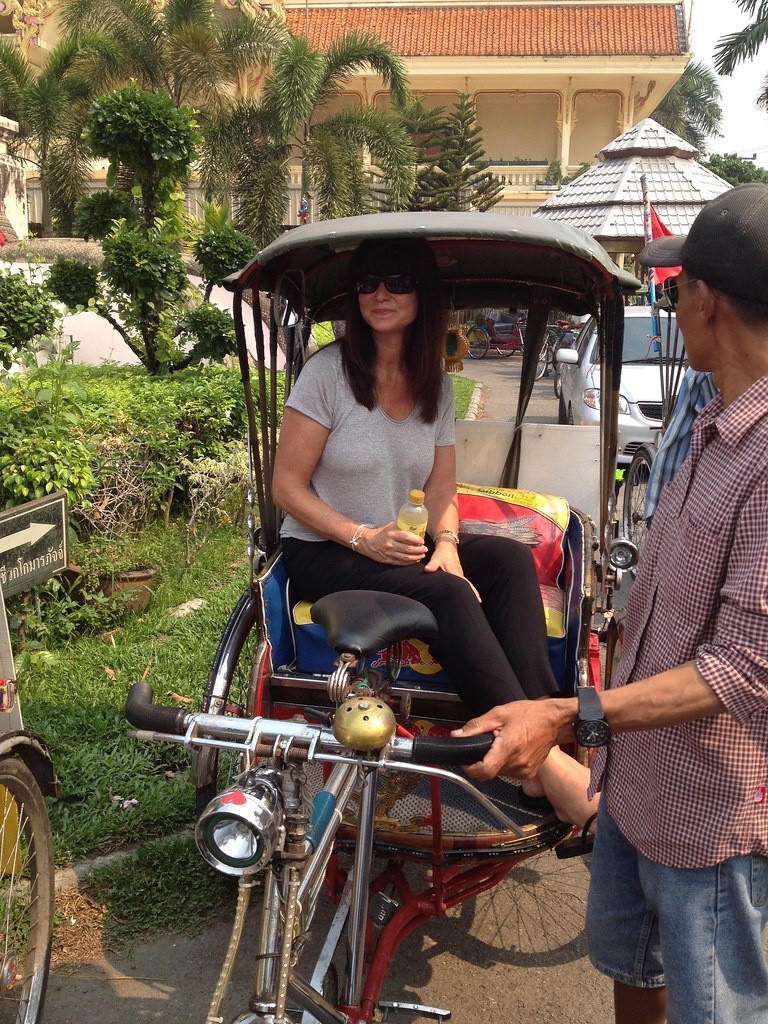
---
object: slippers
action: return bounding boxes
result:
[554,810,598,861]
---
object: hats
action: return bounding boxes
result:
[637,183,768,303]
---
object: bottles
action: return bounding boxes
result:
[394,490,429,542]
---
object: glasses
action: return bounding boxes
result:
[661,276,700,311]
[352,271,419,294]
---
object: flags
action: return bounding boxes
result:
[648,202,686,288]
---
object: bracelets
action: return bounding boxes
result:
[433,530,460,547]
[350,524,367,553]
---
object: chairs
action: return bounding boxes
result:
[253,481,596,705]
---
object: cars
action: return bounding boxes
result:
[553,303,689,470]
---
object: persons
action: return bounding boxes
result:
[556,311,593,344]
[450,182,768,1024]
[271,230,607,861]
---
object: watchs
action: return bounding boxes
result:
[572,686,612,749]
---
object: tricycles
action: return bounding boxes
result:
[115,205,635,1020]
[462,306,579,397]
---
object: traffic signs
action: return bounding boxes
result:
[0,491,71,598]
